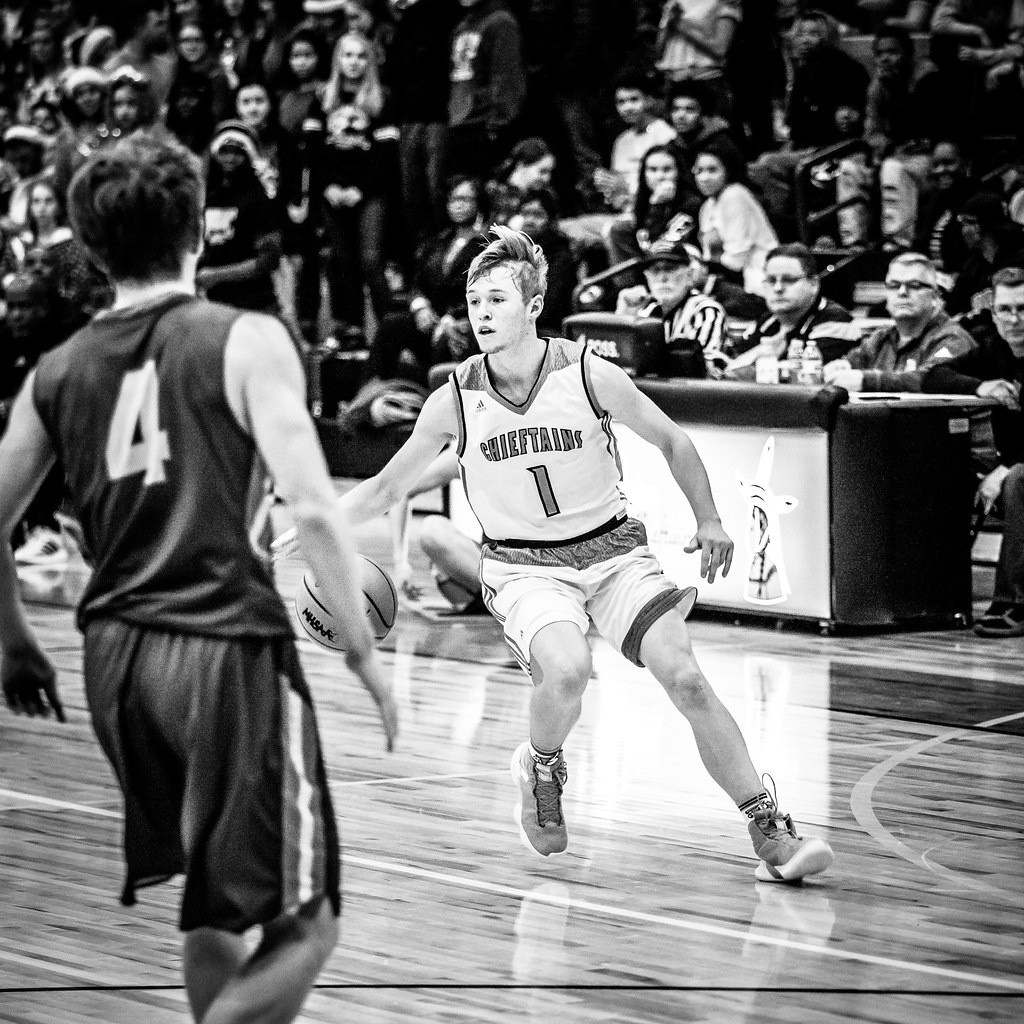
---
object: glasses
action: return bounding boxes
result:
[763,272,807,286]
[991,306,1024,323]
[885,281,934,291]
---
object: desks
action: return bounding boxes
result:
[621,373,1001,634]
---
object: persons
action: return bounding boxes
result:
[0,0,1024,637]
[0,135,398,1023]
[271,224,834,880]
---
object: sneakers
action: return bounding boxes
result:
[514,742,572,857]
[975,601,1024,637]
[16,530,71,565]
[747,771,834,881]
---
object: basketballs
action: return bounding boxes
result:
[295,553,399,653]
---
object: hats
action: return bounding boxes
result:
[642,240,689,267]
